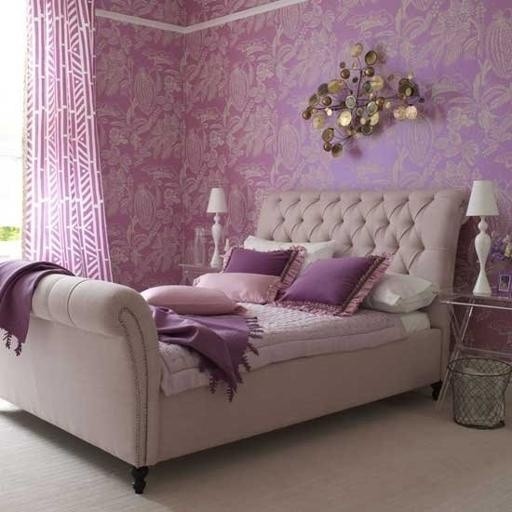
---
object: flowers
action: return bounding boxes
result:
[490,235,512,265]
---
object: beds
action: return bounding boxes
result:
[0,186,472,494]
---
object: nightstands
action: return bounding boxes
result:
[178,263,222,286]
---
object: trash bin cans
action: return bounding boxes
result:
[447,356,512,429]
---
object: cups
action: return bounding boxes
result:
[193,226,205,267]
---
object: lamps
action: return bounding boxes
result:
[465,180,500,298]
[304,42,425,157]
[206,188,228,268]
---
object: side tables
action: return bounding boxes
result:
[434,292,512,415]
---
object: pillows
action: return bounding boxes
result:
[223,247,303,294]
[244,236,336,280]
[139,285,234,314]
[193,272,281,305]
[361,272,439,314]
[277,256,390,315]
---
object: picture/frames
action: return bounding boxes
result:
[499,274,511,292]
